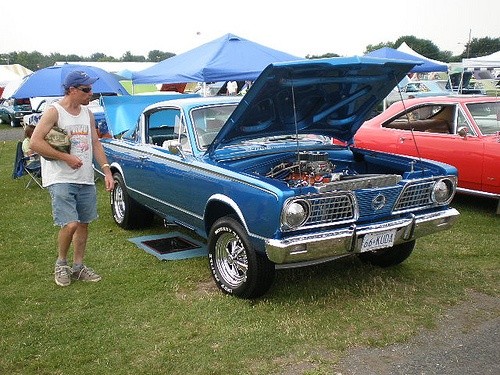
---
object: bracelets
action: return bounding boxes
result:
[102,164,109,168]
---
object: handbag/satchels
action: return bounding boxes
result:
[39,126,71,162]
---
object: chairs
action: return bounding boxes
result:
[12,141,44,191]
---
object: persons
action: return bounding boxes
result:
[21,70,114,285]
[227,80,238,96]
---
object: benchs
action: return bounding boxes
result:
[387,119,452,134]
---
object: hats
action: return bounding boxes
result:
[63,70,99,90]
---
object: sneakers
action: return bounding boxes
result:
[55,262,73,287]
[70,265,102,283]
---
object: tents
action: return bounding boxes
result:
[0,33,500,132]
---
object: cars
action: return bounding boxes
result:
[90,56,458,296]
[332,95,500,201]
[395,71,486,96]
[1,96,35,127]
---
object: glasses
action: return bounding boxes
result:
[74,86,92,93]
